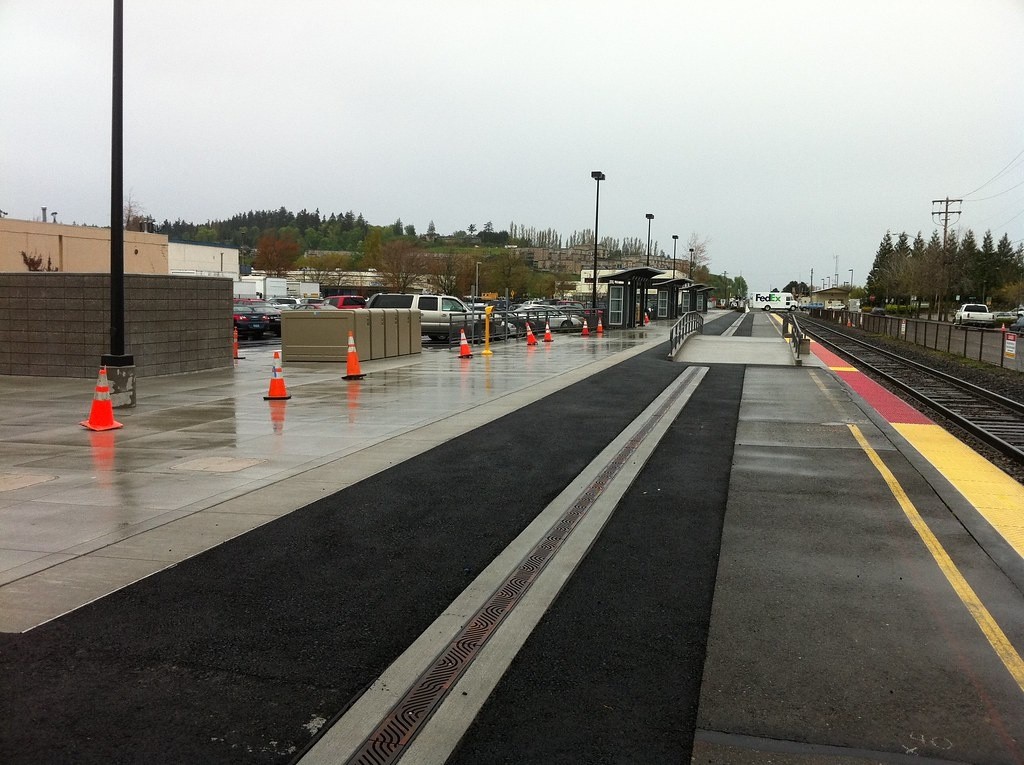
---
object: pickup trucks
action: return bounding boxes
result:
[952,303,996,331]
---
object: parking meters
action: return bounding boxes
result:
[821,278,825,290]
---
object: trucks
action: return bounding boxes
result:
[748,292,798,311]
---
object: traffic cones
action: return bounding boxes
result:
[78,369,124,432]
[458,328,474,358]
[580,319,590,335]
[340,330,367,380]
[542,321,554,342]
[596,317,604,333]
[263,351,292,400]
[525,322,538,345]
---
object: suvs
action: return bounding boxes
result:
[799,302,825,312]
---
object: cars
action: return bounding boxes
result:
[233,291,604,342]
[827,302,847,311]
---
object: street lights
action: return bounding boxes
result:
[475,261,482,301]
[591,170,606,316]
[672,235,679,279]
[835,273,838,288]
[827,277,830,288]
[689,248,694,279]
[644,214,654,308]
[849,268,854,288]
